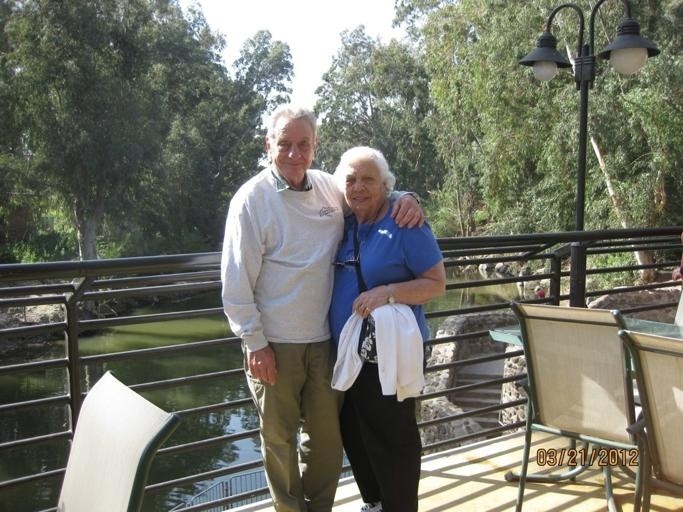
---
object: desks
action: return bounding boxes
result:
[488,315,681,512]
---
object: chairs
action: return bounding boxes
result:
[508,297,681,512]
[37,369,181,512]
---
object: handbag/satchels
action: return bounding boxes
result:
[358,314,377,363]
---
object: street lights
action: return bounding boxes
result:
[517,1,661,306]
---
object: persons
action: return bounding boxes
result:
[217,99,428,512]
[327,145,447,512]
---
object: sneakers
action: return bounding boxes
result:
[361,503,382,512]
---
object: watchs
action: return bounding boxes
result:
[383,285,395,305]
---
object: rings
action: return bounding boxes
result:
[365,306,372,312]
